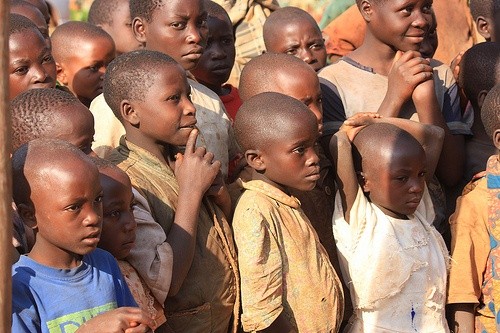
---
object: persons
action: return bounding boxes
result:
[103,49,240,333]
[241,0,500,243]
[9,0,240,262]
[88,156,167,333]
[329,113,452,333]
[232,92,344,333]
[11,138,157,333]
[448,83,500,332]
